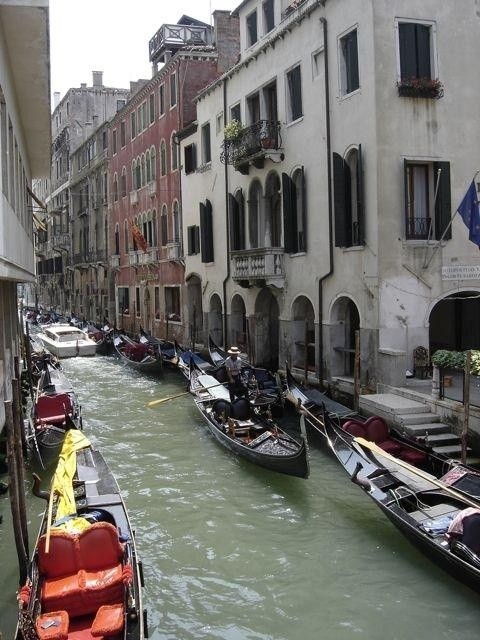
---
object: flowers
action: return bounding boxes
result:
[223,119,243,139]
[401,76,441,87]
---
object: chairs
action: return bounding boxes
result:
[413,345,433,380]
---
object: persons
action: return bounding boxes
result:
[226,347,251,403]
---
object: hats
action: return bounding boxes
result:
[228,347,241,354]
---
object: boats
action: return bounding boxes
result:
[8,427,149,640]
[318,400,479,598]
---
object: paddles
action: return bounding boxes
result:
[44,474,55,554]
[285,390,324,425]
[36,378,41,401]
[172,358,189,372]
[149,382,228,405]
[354,436,479,509]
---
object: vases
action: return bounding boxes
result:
[231,137,242,145]
[402,88,435,97]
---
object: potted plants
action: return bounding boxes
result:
[260,123,277,148]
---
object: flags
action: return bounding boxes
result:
[457,179,480,250]
